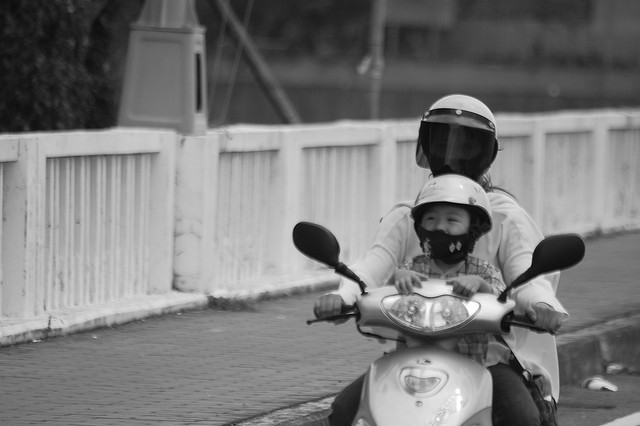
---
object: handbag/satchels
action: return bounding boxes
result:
[522,373,558,426]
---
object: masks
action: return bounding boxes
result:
[413,222,475,265]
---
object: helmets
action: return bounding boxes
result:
[411,173,493,234]
[415,95,497,176]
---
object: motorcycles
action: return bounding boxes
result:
[292,222,585,425]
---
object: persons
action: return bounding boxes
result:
[386,174,506,298]
[314,93,571,425]
